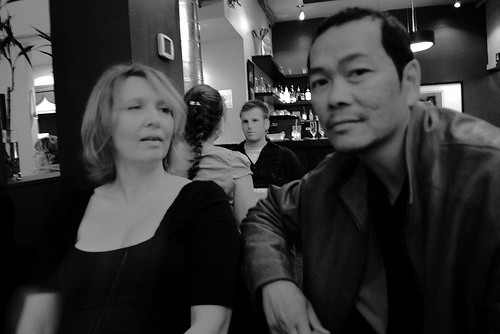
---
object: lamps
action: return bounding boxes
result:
[405,0,435,53]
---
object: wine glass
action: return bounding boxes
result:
[318,121,326,139]
[309,121,317,140]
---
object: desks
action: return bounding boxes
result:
[0,172,61,266]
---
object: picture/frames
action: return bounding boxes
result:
[419,81,465,114]
[246,59,255,87]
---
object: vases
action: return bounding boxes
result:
[1,128,20,176]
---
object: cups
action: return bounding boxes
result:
[292,126,301,141]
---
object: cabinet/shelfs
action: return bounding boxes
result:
[252,55,300,121]
[274,74,320,124]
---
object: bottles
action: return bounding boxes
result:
[302,107,307,120]
[309,109,313,120]
[255,76,311,103]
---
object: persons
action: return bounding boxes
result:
[16,63,247,334]
[239,6,500,334]
[230,99,289,188]
[164,84,259,230]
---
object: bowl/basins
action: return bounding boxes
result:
[268,133,285,141]
